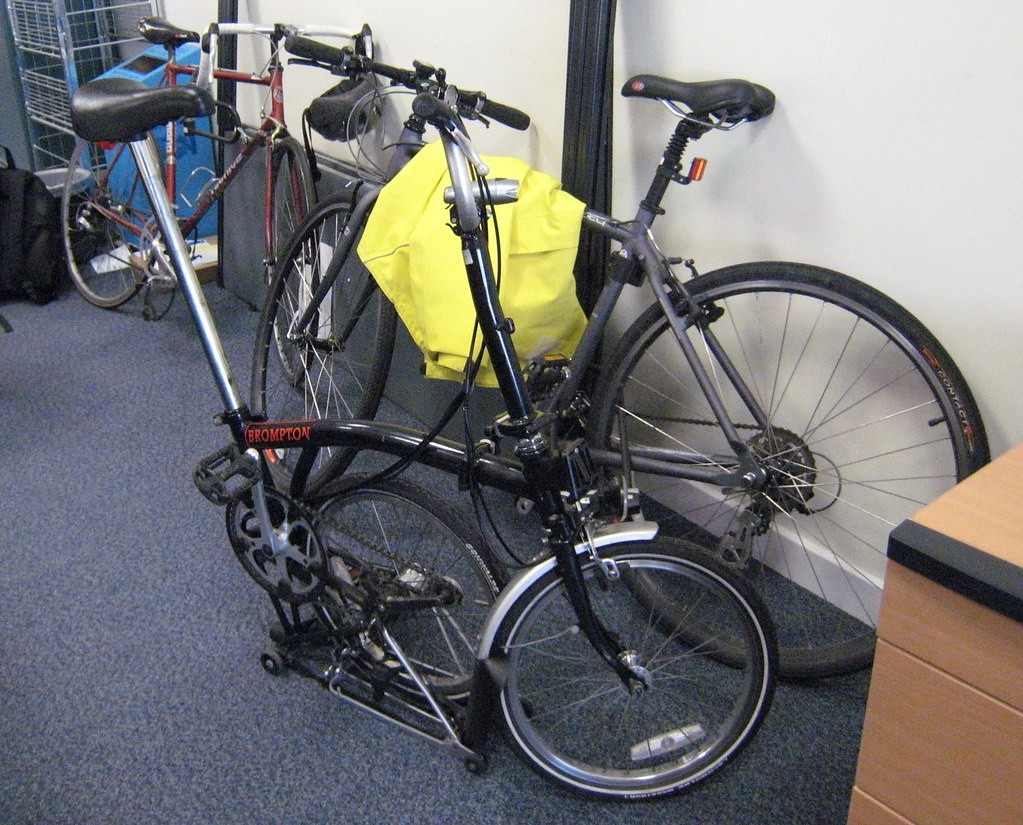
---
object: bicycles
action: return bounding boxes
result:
[69,78,779,804]
[60,15,374,386]
[251,35,992,681]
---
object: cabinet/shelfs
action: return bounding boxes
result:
[847,443,1023,825]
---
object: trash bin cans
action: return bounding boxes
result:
[86,43,220,294]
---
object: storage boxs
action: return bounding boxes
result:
[128,235,219,294]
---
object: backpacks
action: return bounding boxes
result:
[0,167,71,306]
